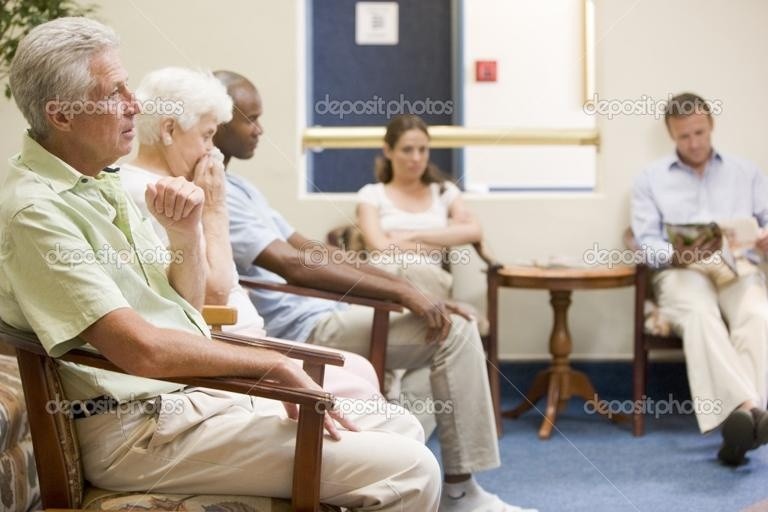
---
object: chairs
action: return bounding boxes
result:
[232,273,407,408]
[329,225,502,434]
[201,305,239,335]
[0,319,346,511]
[624,225,731,438]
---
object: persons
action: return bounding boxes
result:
[212,66,533,512]
[1,17,443,511]
[115,66,385,405]
[624,91,768,466]
[351,111,492,342]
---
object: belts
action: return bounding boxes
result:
[70,386,189,418]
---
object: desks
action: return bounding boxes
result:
[496,264,638,439]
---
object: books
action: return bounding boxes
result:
[659,216,763,278]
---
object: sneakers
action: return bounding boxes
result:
[438,492,538,512]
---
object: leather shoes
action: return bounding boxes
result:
[718,408,754,468]
[746,405,767,451]
